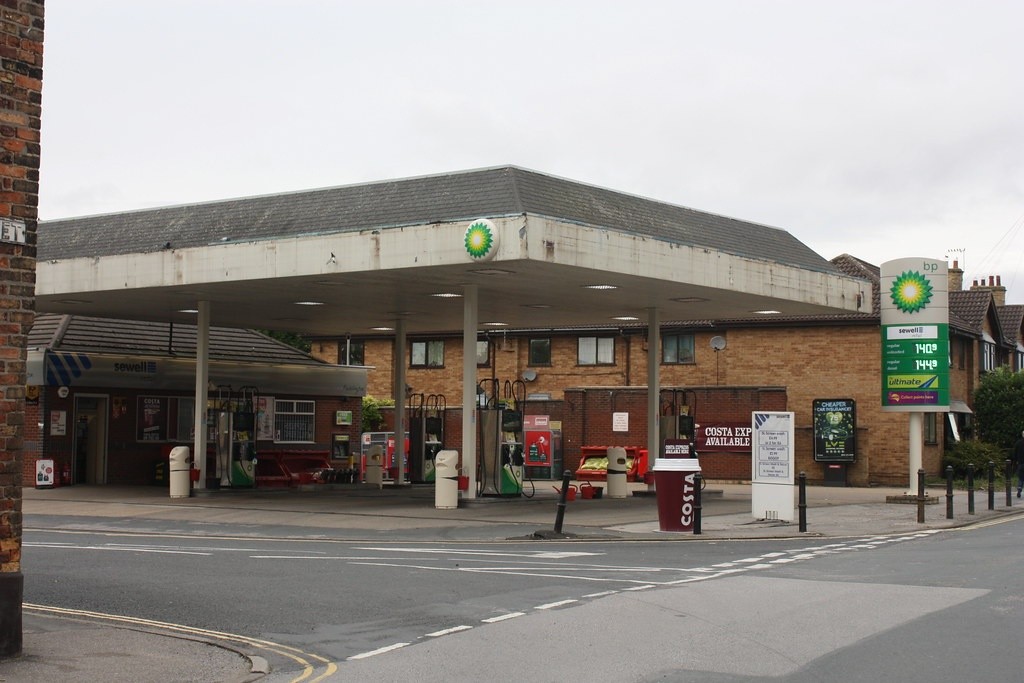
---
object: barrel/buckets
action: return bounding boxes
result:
[592,487,603,499]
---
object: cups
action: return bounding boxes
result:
[44,473,48,481]
[652,458,702,531]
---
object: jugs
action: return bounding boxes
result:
[579,483,599,499]
[298,469,315,484]
[552,484,578,501]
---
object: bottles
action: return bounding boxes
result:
[38,471,43,481]
[319,467,361,484]
[529,441,538,461]
[540,453,547,462]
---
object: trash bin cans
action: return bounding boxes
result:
[366,445,382,491]
[434,450,459,509]
[169,446,190,498]
[606,448,627,499]
